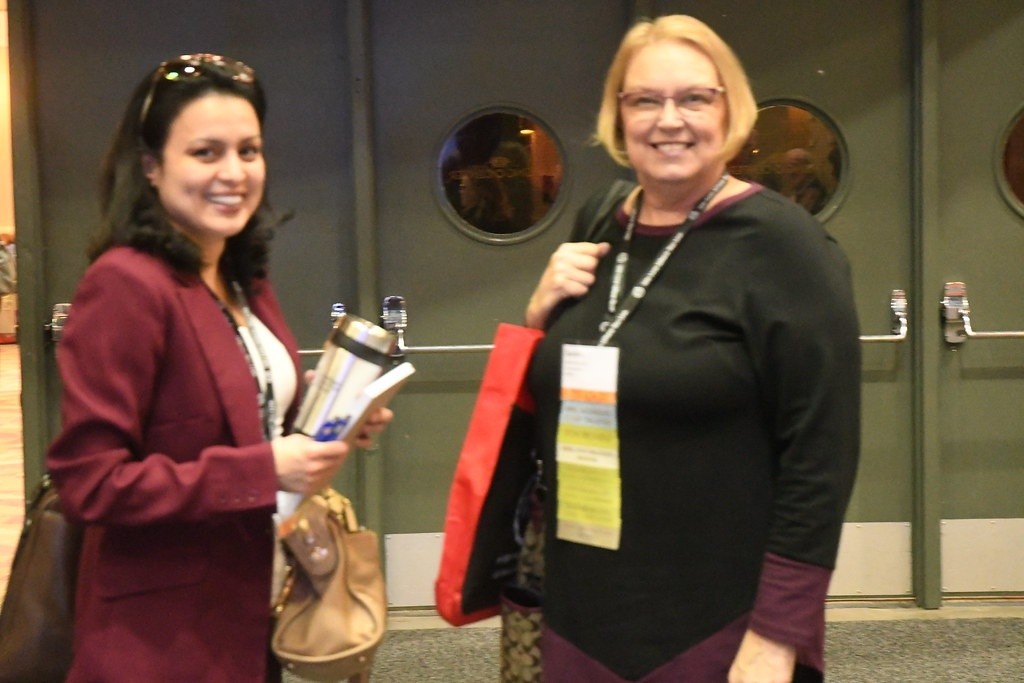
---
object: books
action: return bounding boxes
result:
[334,360,418,442]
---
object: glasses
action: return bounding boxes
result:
[138,54,255,150]
[616,85,726,113]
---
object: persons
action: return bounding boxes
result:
[440,113,564,236]
[521,13,863,683]
[737,145,841,217]
[43,53,396,683]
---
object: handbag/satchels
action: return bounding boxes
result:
[496,447,543,681]
[2,473,82,683]
[271,485,386,682]
[435,320,547,628]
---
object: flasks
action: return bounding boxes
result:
[291,313,397,442]
